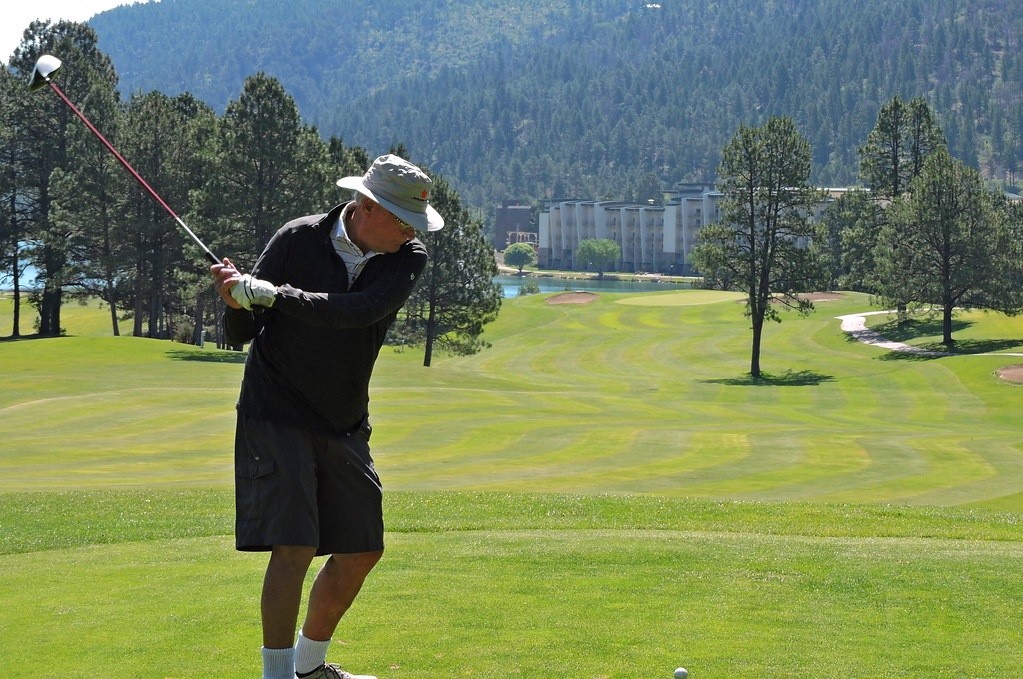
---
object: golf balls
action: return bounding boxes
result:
[673,668,688,679]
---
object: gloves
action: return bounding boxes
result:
[230,272,276,311]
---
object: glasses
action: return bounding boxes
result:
[389,211,412,229]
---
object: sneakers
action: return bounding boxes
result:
[295,661,377,679]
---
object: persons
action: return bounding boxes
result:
[210,154,445,679]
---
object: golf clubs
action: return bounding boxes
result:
[27,53,224,265]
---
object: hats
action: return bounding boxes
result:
[336,154,447,232]
